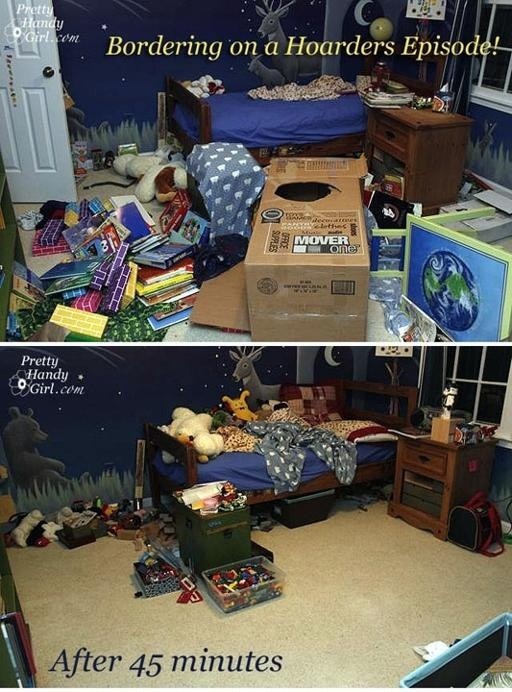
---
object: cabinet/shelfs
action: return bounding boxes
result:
[0,151,27,342]
[0,533,32,688]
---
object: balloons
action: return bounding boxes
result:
[385,172,406,202]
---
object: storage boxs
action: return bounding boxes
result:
[439,190,512,242]
[189,151,372,341]
[133,539,185,598]
[201,556,287,613]
[269,489,334,529]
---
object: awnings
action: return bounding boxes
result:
[206,559,287,613]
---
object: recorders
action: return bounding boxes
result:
[410,405,472,432]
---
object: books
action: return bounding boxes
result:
[38,194,209,331]
[387,424,431,441]
[357,87,415,110]
[397,294,456,342]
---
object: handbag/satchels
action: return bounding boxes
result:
[447,492,503,552]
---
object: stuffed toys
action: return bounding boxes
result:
[217,481,248,512]
[156,389,259,465]
[110,146,189,201]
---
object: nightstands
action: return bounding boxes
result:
[388,429,500,542]
[366,100,475,214]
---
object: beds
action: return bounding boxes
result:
[145,381,418,507]
[164,75,366,150]
